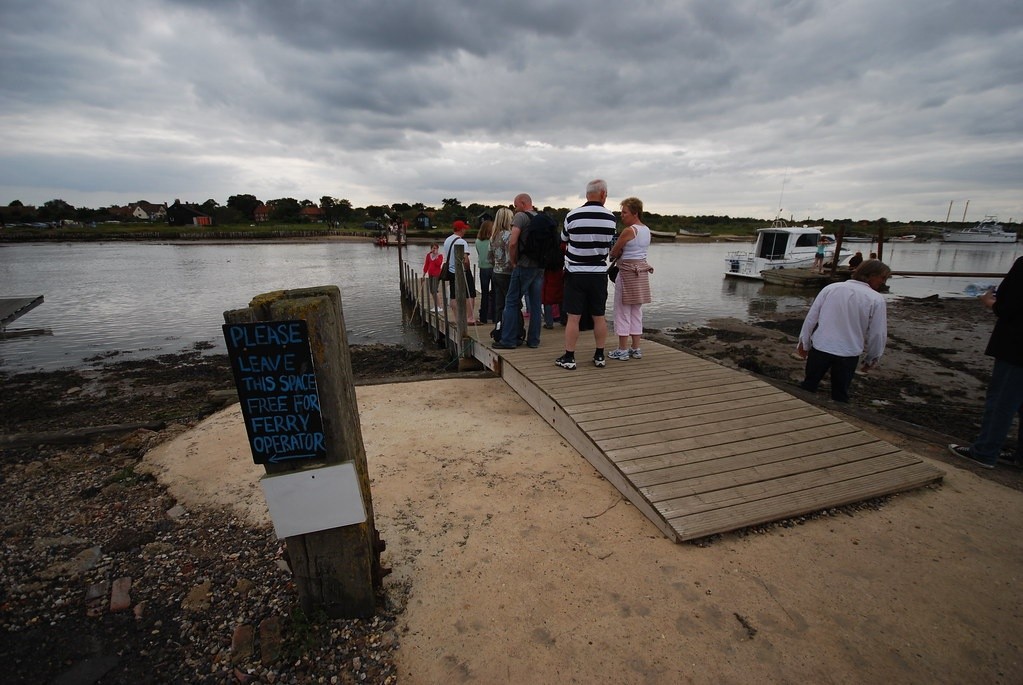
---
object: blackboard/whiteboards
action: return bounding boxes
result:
[223,319,327,464]
[258,459,368,539]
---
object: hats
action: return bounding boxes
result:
[453,220,469,230]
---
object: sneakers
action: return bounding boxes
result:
[629,347,642,359]
[593,354,606,367]
[607,348,628,361]
[555,354,577,369]
[948,444,995,469]
[997,451,1023,469]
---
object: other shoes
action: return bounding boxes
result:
[492,342,516,349]
[429,307,443,313]
[470,320,484,326]
[527,340,539,347]
[543,325,553,329]
[553,317,561,322]
[818,272,825,275]
[522,311,530,317]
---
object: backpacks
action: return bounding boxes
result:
[490,308,526,346]
[518,212,561,268]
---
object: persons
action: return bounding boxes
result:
[811,236,835,274]
[491,194,545,349]
[379,222,399,247]
[554,180,617,370]
[848,253,877,269]
[948,257,1023,472]
[797,260,894,403]
[607,198,654,362]
[423,193,569,330]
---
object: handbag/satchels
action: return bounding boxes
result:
[438,263,452,281]
[607,258,619,282]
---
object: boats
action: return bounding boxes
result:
[680,229,711,237]
[942,215,1017,243]
[843,237,873,243]
[888,235,916,242]
[650,230,677,238]
[724,219,857,283]
